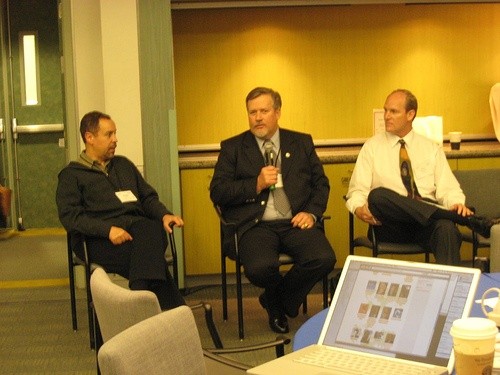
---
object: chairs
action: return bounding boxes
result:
[214,203,331,342]
[67,228,179,352]
[98,306,255,375]
[343,194,431,262]
[90,268,224,350]
[457,207,490,269]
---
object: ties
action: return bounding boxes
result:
[398,140,422,201]
[264,140,291,216]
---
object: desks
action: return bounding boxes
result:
[293,271,500,351]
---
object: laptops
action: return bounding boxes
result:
[246,255,482,375]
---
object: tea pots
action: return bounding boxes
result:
[481,287,500,328]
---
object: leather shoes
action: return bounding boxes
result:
[467,208,494,239]
[259,291,289,334]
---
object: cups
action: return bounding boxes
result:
[450,316,499,375]
[449,132,462,150]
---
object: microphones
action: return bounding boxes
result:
[265,142,275,191]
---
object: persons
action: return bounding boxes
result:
[209,86,336,334]
[56,111,186,313]
[344,89,494,266]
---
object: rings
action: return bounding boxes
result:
[304,223,308,228]
